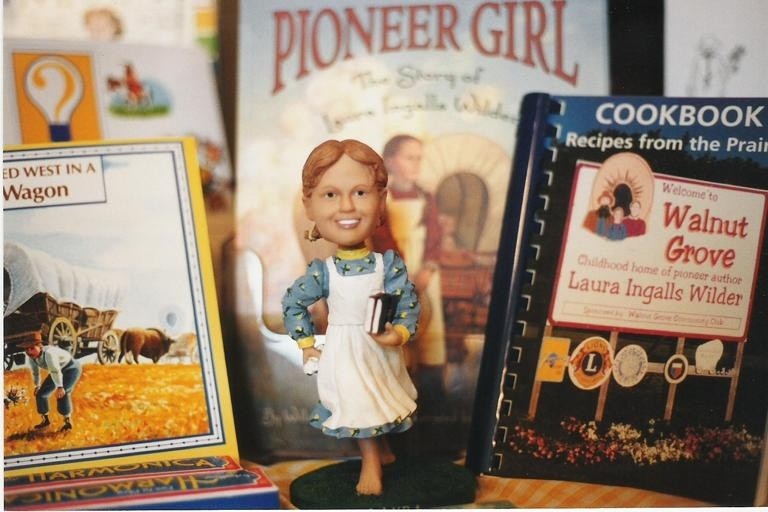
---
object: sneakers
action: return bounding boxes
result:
[35,420,51,429]
[61,423,72,432]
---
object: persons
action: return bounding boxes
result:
[15,331,83,433]
[382,132,447,368]
[585,190,646,240]
[281,137,424,499]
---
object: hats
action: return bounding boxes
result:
[598,192,613,204]
[15,333,44,347]
